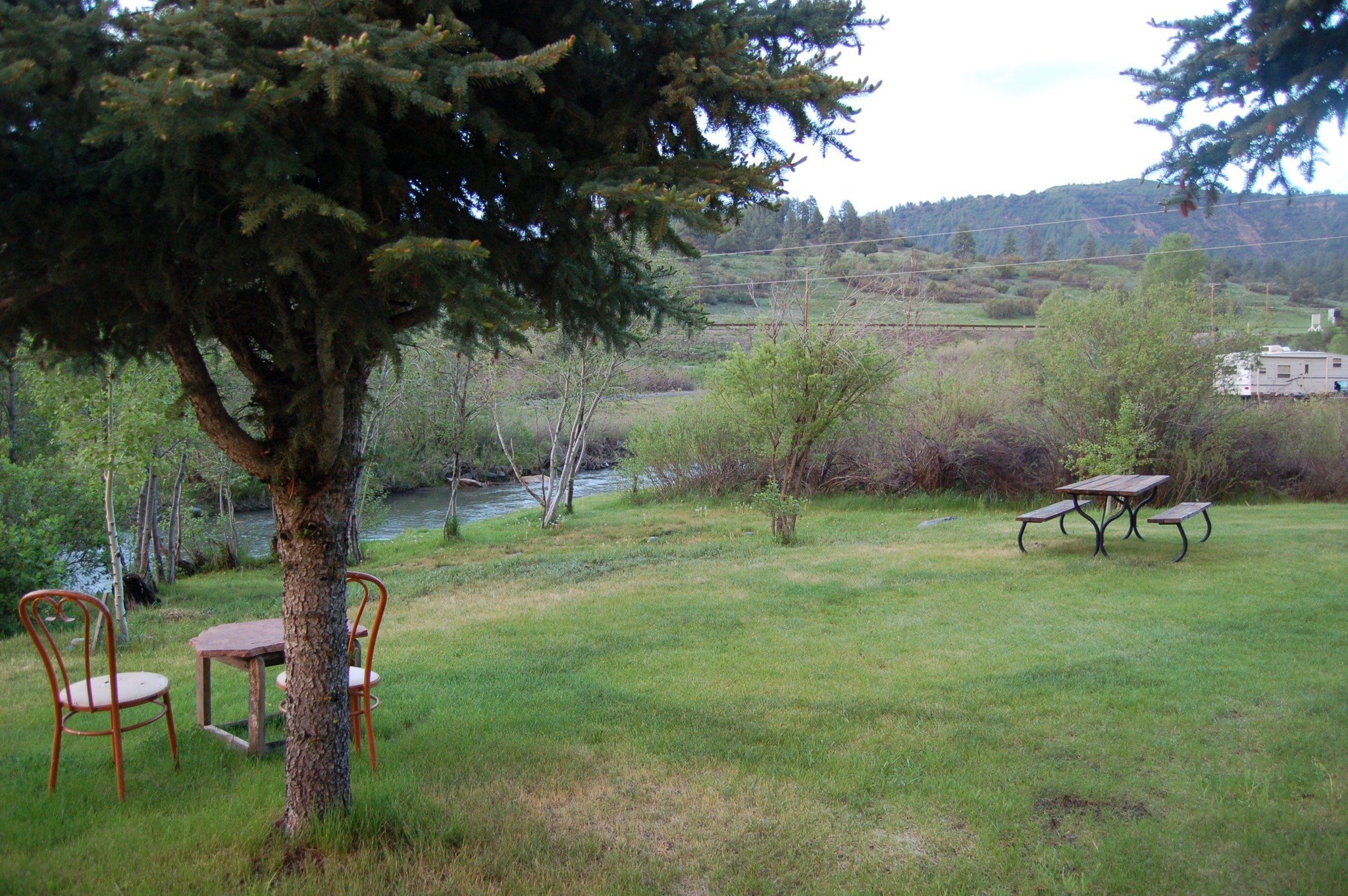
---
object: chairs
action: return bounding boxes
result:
[17,588,181,806]
[275,570,388,782]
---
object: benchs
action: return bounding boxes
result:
[1147,502,1212,562]
[1015,499,1092,555]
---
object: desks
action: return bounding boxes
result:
[1056,474,1170,561]
[187,618,369,762]
